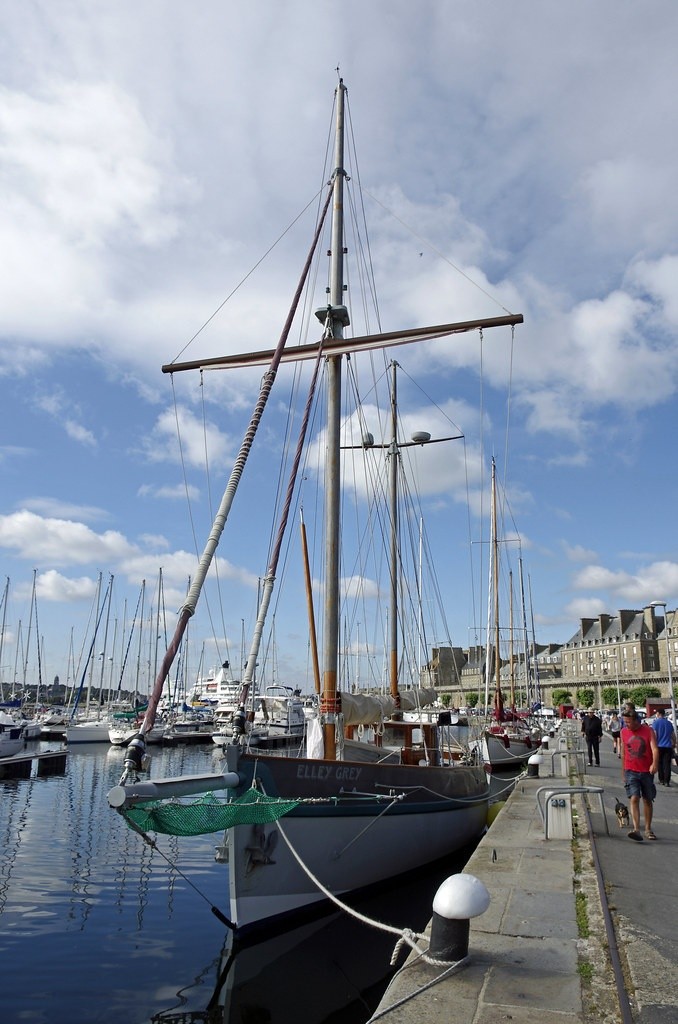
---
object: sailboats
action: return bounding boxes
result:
[104,77,525,926]
[0,312,554,790]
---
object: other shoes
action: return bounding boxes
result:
[661,782,669,787]
[614,747,616,753]
[594,764,600,767]
[617,754,621,759]
[586,762,592,766]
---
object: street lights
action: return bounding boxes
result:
[608,655,622,714]
[650,600,678,740]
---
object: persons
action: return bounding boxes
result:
[608,711,622,759]
[582,709,603,767]
[620,710,659,841]
[652,709,676,786]
[580,710,585,721]
[621,703,641,728]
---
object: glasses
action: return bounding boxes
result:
[622,713,632,717]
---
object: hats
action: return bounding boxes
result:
[585,707,596,713]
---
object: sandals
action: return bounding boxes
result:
[644,830,657,840]
[628,830,644,841]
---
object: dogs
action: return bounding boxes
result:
[615,797,629,828]
